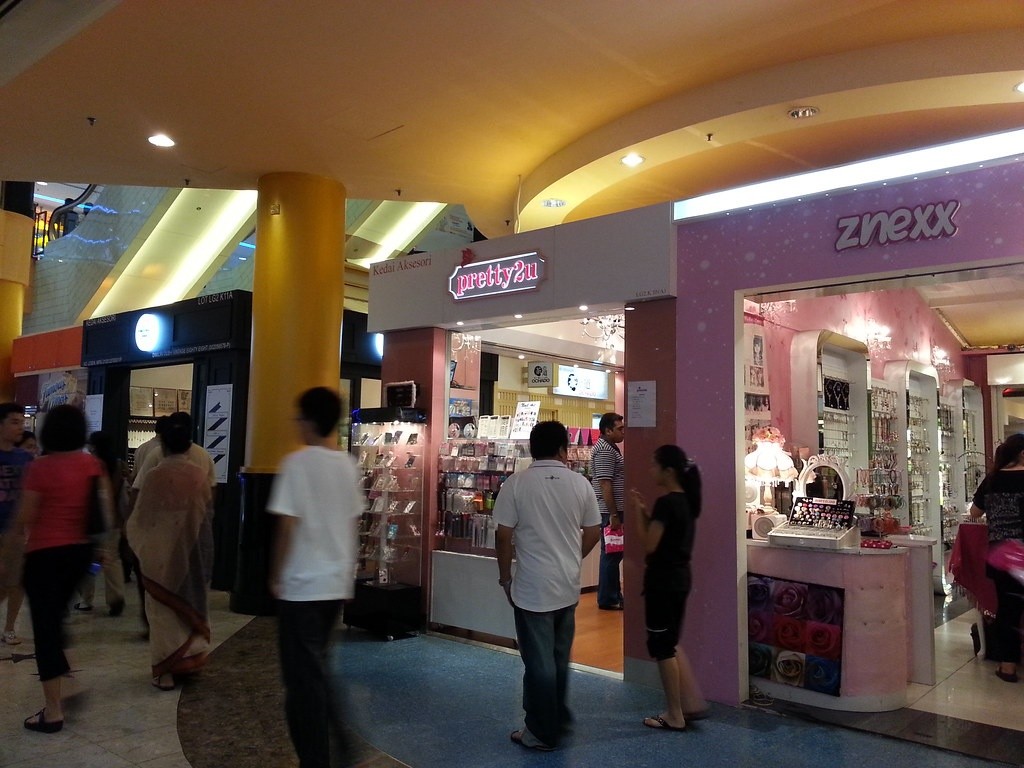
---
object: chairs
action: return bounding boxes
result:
[948,519,1024,665]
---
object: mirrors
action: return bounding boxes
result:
[383,380,421,409]
[792,452,852,501]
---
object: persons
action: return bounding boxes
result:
[632,444,710,732]
[13,404,116,733]
[0,402,217,646]
[495,420,602,750]
[754,338,763,366]
[970,434,1024,683]
[591,413,624,610]
[806,468,844,499]
[63,198,79,237]
[122,417,213,691]
[267,386,365,768]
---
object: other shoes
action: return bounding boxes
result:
[74,603,92,611]
[109,600,125,617]
[1,630,21,646]
[511,729,557,751]
[597,591,624,610]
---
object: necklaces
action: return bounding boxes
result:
[938,403,954,435]
[822,376,855,468]
[906,395,930,536]
[873,388,897,468]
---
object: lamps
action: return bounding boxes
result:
[744,426,799,516]
[451,333,482,363]
[580,314,624,354]
[931,345,950,366]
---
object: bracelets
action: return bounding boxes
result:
[498,577,512,586]
[856,468,907,533]
[610,512,618,517]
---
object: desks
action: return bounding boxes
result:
[748,533,937,714]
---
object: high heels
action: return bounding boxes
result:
[24,707,64,733]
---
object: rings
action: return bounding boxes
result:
[791,498,850,530]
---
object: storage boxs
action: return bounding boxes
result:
[448,398,472,417]
[449,416,474,430]
[767,495,859,550]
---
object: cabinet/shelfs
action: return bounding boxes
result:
[565,444,595,484]
[342,406,429,642]
[941,378,986,524]
[939,395,960,595]
[871,378,902,528]
[788,327,870,535]
[449,340,480,430]
[883,360,944,596]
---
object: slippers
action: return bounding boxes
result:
[681,705,711,721]
[995,664,1018,683]
[643,714,686,732]
[152,675,182,691]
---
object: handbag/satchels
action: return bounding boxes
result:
[603,523,624,555]
